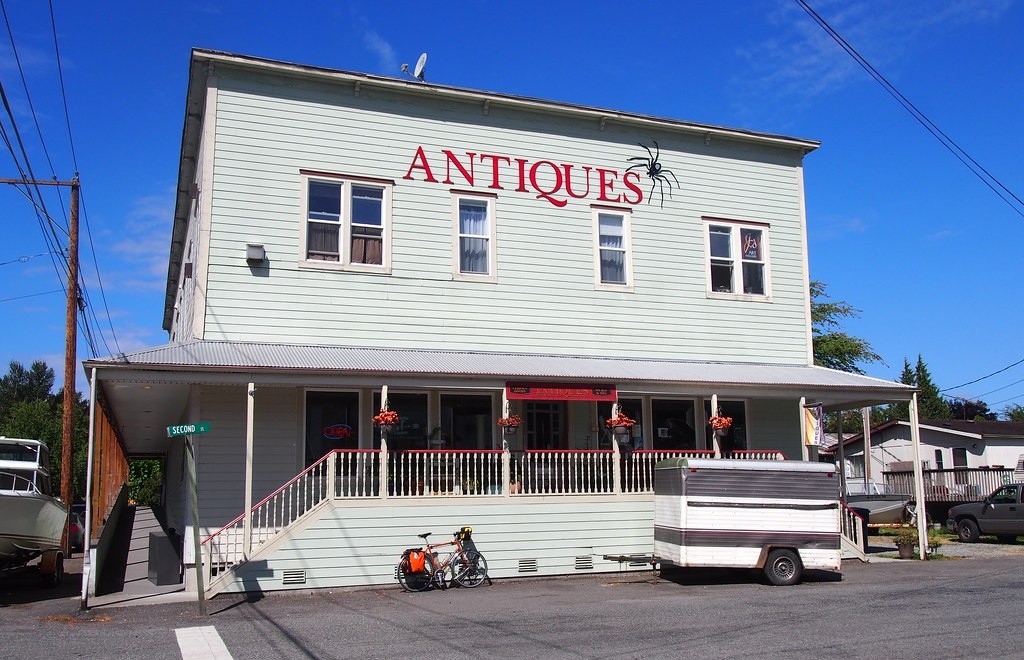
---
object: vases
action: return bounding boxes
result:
[613,425,627,435]
[504,425,518,435]
[380,423,395,432]
[716,427,728,436]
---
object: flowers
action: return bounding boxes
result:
[606,412,636,428]
[372,407,400,425]
[709,416,733,429]
[497,417,524,426]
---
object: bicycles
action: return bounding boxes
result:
[902,502,933,527]
[397,527,487,592]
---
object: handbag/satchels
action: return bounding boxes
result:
[409,551,426,574]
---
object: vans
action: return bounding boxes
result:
[72,504,86,513]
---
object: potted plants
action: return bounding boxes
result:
[893,531,918,559]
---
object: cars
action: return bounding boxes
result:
[70,512,84,549]
[81,511,86,524]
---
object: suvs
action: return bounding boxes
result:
[946,483,1024,543]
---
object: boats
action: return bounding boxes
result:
[845,479,911,526]
[0,437,68,568]
